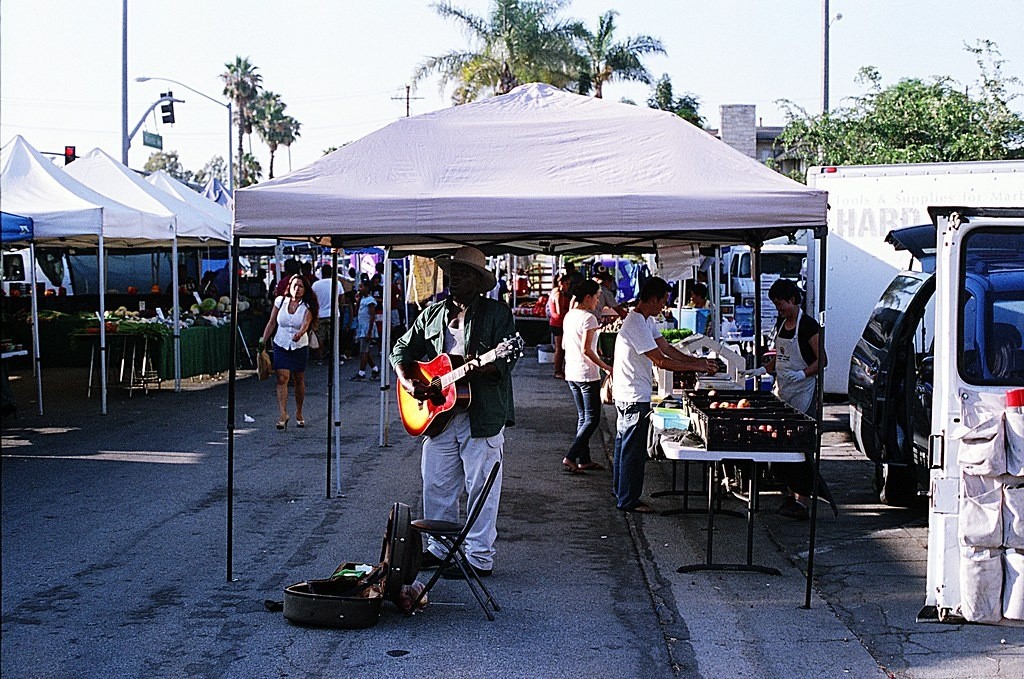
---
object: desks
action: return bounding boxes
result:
[650,434,808,576]
[68,311,271,399]
[1,349,28,419]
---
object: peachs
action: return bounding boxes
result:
[689,389,793,437]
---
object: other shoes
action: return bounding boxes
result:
[554,371,565,380]
[767,496,809,520]
[348,374,367,382]
[442,558,492,579]
[419,549,444,567]
[368,370,381,381]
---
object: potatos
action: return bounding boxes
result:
[115,306,139,320]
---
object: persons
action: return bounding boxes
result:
[269,258,319,379]
[737,277,826,518]
[685,283,715,309]
[388,246,517,578]
[166,264,197,294]
[297,259,345,365]
[592,272,628,323]
[201,261,268,298]
[262,273,312,430]
[548,272,570,379]
[340,262,401,382]
[562,279,612,475]
[518,268,534,294]
[611,277,719,513]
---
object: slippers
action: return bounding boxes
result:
[561,465,585,476]
[617,502,656,514]
[577,463,604,470]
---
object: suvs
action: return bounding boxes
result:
[848,246,1024,502]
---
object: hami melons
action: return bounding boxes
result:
[217,296,249,312]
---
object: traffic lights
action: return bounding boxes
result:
[160,92,175,124]
[65,146,76,166]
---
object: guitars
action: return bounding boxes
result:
[396,331,526,439]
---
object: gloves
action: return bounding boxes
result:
[738,366,767,379]
[782,369,806,384]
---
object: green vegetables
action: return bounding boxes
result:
[18,310,173,344]
[661,328,694,344]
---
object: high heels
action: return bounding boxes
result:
[295,412,305,428]
[275,414,289,432]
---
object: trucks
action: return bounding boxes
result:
[720,161,1024,403]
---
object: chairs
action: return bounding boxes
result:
[406,460,500,621]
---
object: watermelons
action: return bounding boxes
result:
[190,298,216,313]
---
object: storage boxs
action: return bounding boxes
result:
[668,307,710,334]
[745,373,771,391]
[682,389,817,452]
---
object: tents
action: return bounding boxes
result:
[225,86,828,609]
[1,134,233,414]
[200,177,280,285]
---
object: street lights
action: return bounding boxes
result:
[823,0,842,109]
[136,77,233,197]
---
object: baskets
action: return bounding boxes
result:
[681,388,819,453]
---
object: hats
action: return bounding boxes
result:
[435,247,497,294]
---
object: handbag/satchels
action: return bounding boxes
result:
[546,291,557,318]
[600,373,613,405]
[257,348,271,381]
[309,329,320,349]
